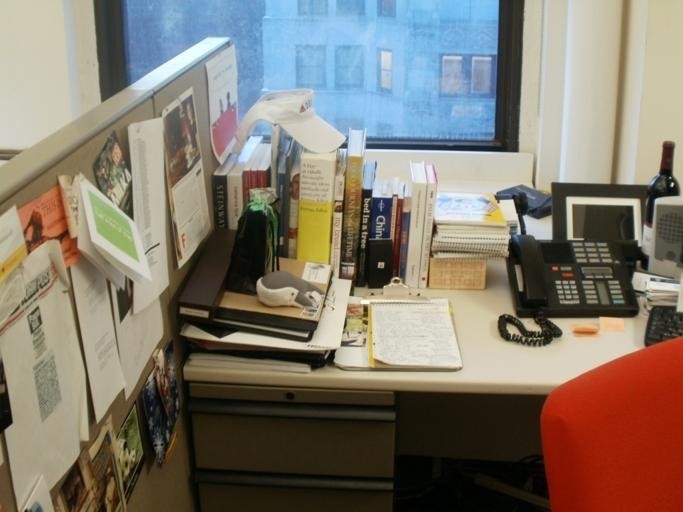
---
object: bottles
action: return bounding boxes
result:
[641,140,679,270]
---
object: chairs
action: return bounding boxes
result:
[539,335,683,512]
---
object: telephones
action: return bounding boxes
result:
[507,235,641,317]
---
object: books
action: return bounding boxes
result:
[179,106,517,373]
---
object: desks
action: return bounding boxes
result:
[181,257,650,511]
